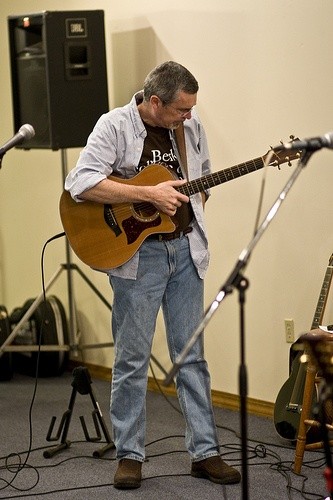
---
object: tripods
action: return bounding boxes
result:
[0,146,177,390]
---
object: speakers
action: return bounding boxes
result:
[6,9,110,152]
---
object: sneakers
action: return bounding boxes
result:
[191,456,241,485]
[113,458,142,488]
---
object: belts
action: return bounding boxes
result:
[144,226,193,242]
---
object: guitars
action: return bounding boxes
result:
[58,135,308,272]
[274,252,333,442]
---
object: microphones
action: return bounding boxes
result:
[0,122,36,159]
[271,131,333,151]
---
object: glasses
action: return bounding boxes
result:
[161,99,192,118]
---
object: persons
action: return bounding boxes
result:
[65,63,242,487]
